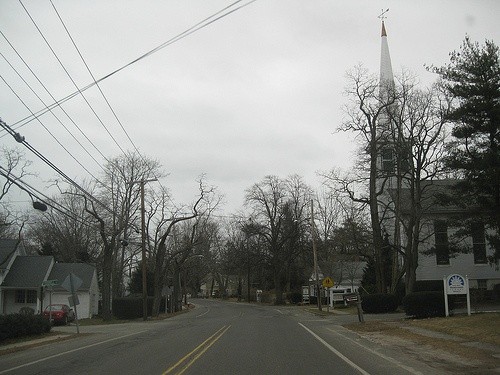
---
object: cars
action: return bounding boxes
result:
[44,304,75,325]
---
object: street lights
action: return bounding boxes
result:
[183,255,204,305]
